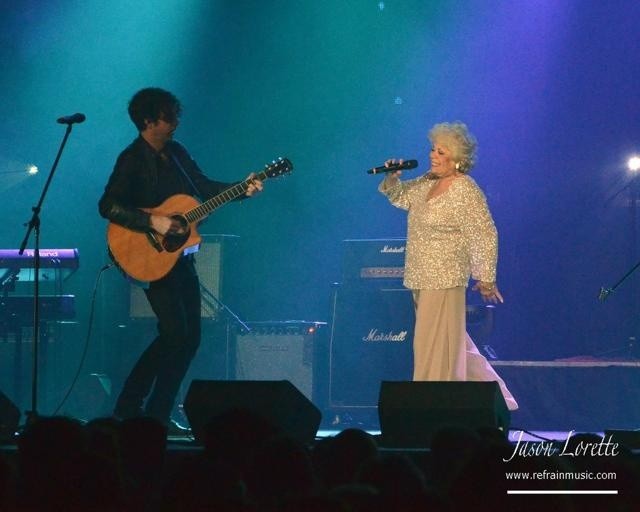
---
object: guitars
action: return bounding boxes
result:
[107,157,292,288]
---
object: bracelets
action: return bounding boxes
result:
[479,280,496,291]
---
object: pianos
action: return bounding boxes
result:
[0,248,80,326]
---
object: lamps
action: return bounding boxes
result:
[375,377,512,450]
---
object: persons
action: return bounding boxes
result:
[366,119,518,411]
[98,89,264,432]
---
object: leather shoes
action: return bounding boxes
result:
[114,412,145,422]
[154,418,193,436]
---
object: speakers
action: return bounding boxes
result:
[378,381,511,438]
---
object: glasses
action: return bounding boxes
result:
[153,112,177,125]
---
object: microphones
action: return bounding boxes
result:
[367,159,419,174]
[58,113,87,124]
